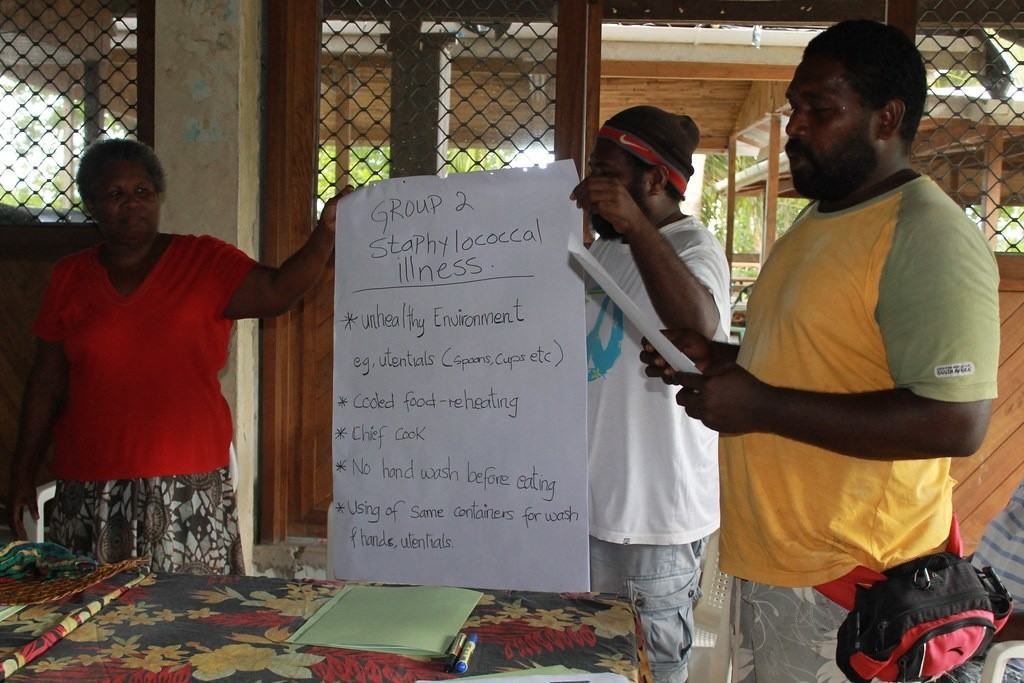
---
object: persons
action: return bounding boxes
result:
[10,139,354,578]
[637,17,1001,683]
[570,104,731,682]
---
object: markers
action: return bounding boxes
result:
[444,632,478,673]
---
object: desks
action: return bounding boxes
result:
[0,561,656,683]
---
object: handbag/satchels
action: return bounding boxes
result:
[836,552,1015,683]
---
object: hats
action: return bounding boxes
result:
[597,106,700,197]
